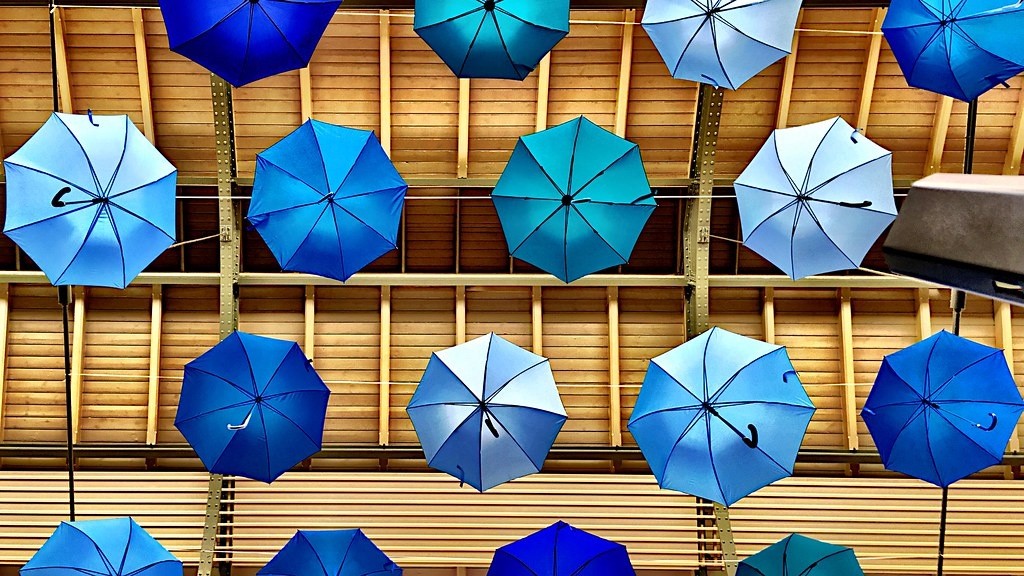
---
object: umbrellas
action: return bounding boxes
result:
[858,329,1024,491]
[641,0,804,91]
[159,0,344,87]
[881,0,1023,103]
[627,325,818,508]
[244,117,408,285]
[411,0,574,82]
[19,516,184,576]
[1,108,177,290]
[256,528,403,576]
[405,331,569,492]
[735,533,865,576]
[486,520,637,576]
[488,115,660,285]
[734,114,898,282]
[173,329,331,485]
